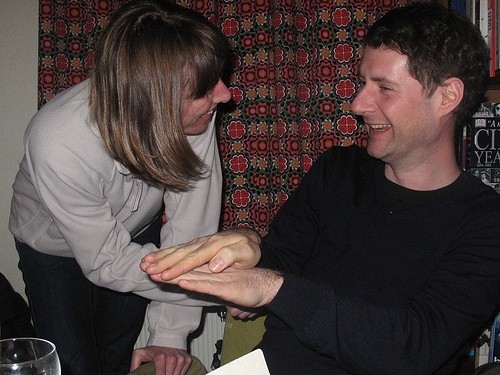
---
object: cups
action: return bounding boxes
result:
[0,338,61,375]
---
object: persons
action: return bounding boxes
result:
[141,4,500,374]
[8,1,232,375]
